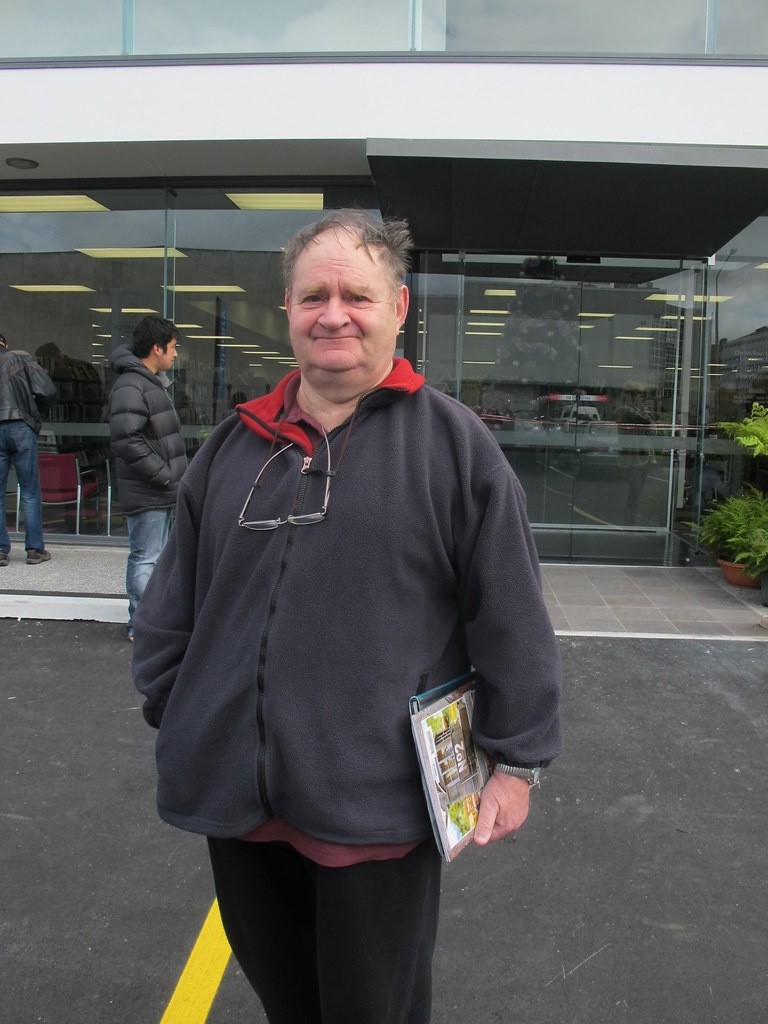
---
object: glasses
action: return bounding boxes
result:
[239,426,332,531]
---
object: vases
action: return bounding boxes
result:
[680,480,768,588]
[726,526,768,608]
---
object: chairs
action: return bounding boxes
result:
[15,452,100,534]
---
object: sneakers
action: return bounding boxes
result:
[0,553,10,566]
[26,549,51,564]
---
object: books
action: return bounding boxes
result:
[408,668,540,863]
[36,356,110,443]
[179,359,215,424]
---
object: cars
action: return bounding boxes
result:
[478,393,658,477]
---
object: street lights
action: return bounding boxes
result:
[714,247,740,423]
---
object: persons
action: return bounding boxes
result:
[180,395,200,452]
[616,380,657,526]
[34,342,107,535]
[133,210,561,1024]
[0,335,56,566]
[223,392,247,419]
[107,315,188,640]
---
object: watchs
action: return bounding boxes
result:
[494,763,540,785]
